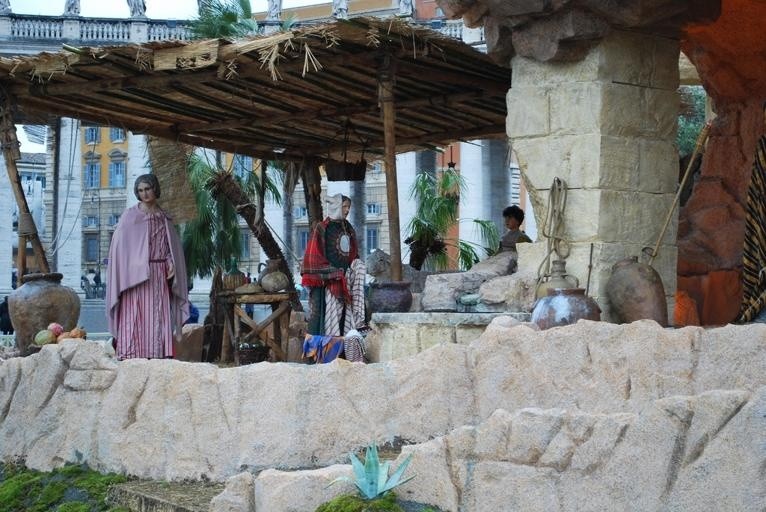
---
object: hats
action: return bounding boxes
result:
[323,192,343,223]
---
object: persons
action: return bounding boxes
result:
[104,173,186,361]
[300,193,377,362]
[495,204,532,258]
[182,301,200,325]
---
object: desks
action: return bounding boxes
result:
[218,291,290,365]
